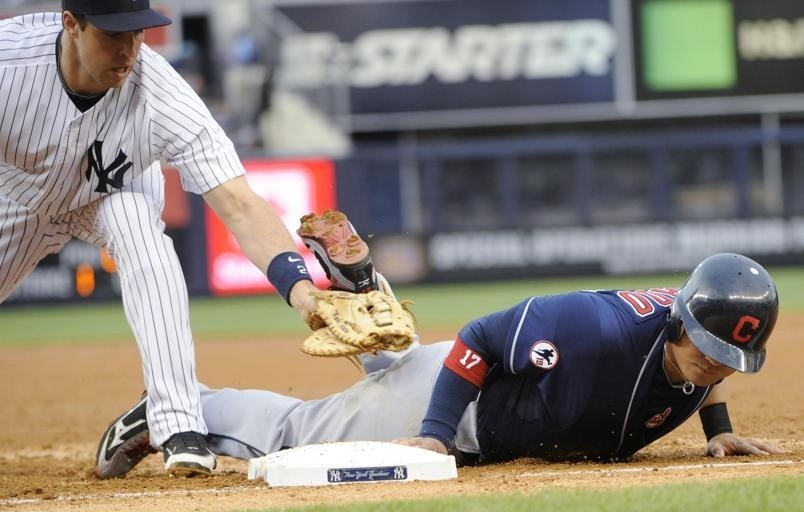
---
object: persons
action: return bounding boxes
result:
[94,207,793,480]
[0,0,417,477]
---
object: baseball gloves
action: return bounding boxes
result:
[300,288,414,356]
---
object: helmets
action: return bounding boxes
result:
[677,254,779,374]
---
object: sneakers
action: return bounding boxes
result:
[93,391,156,477]
[161,430,217,476]
[294,209,376,294]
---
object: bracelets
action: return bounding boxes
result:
[267,248,314,306]
[694,399,733,442]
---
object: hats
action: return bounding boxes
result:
[61,0,172,35]
